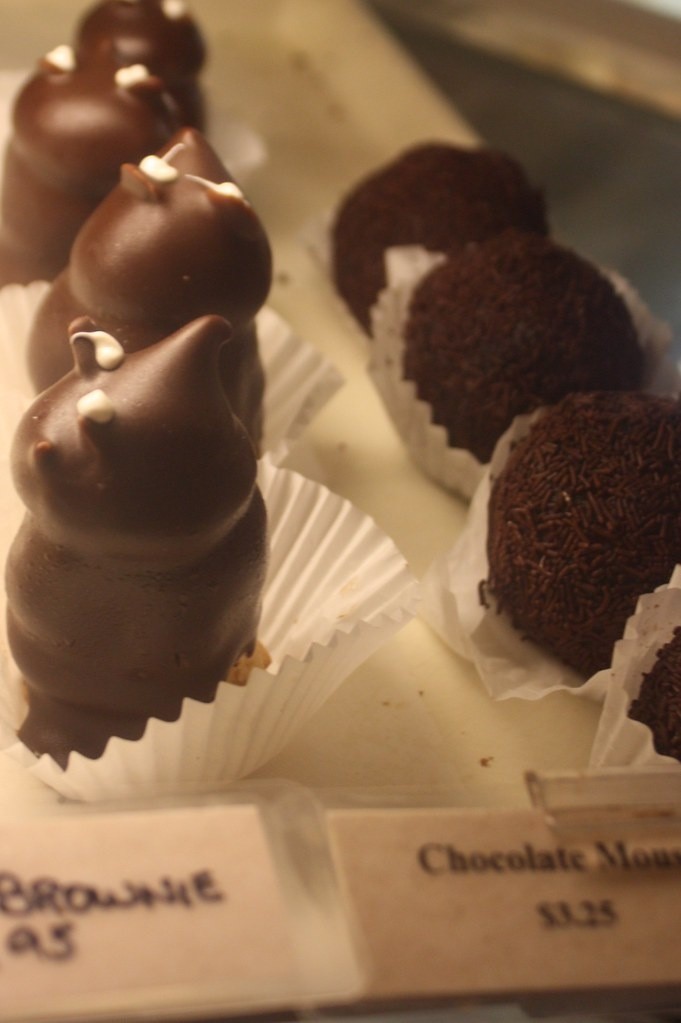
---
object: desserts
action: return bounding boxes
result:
[0,0,681,805]
